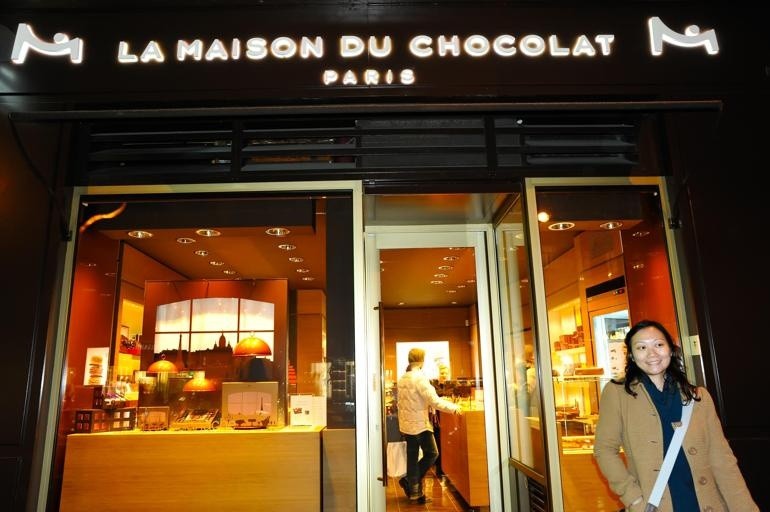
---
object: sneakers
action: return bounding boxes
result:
[410,491,424,500]
[398,477,409,495]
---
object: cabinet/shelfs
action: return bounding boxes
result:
[548,298,601,423]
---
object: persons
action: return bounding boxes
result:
[405,362,442,468]
[394,346,464,505]
[590,318,762,512]
[514,340,541,412]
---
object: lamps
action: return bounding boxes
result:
[233,330,272,356]
[183,369,215,391]
[147,353,179,374]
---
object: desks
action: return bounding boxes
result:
[59,426,324,511]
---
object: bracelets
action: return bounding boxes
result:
[630,496,644,506]
